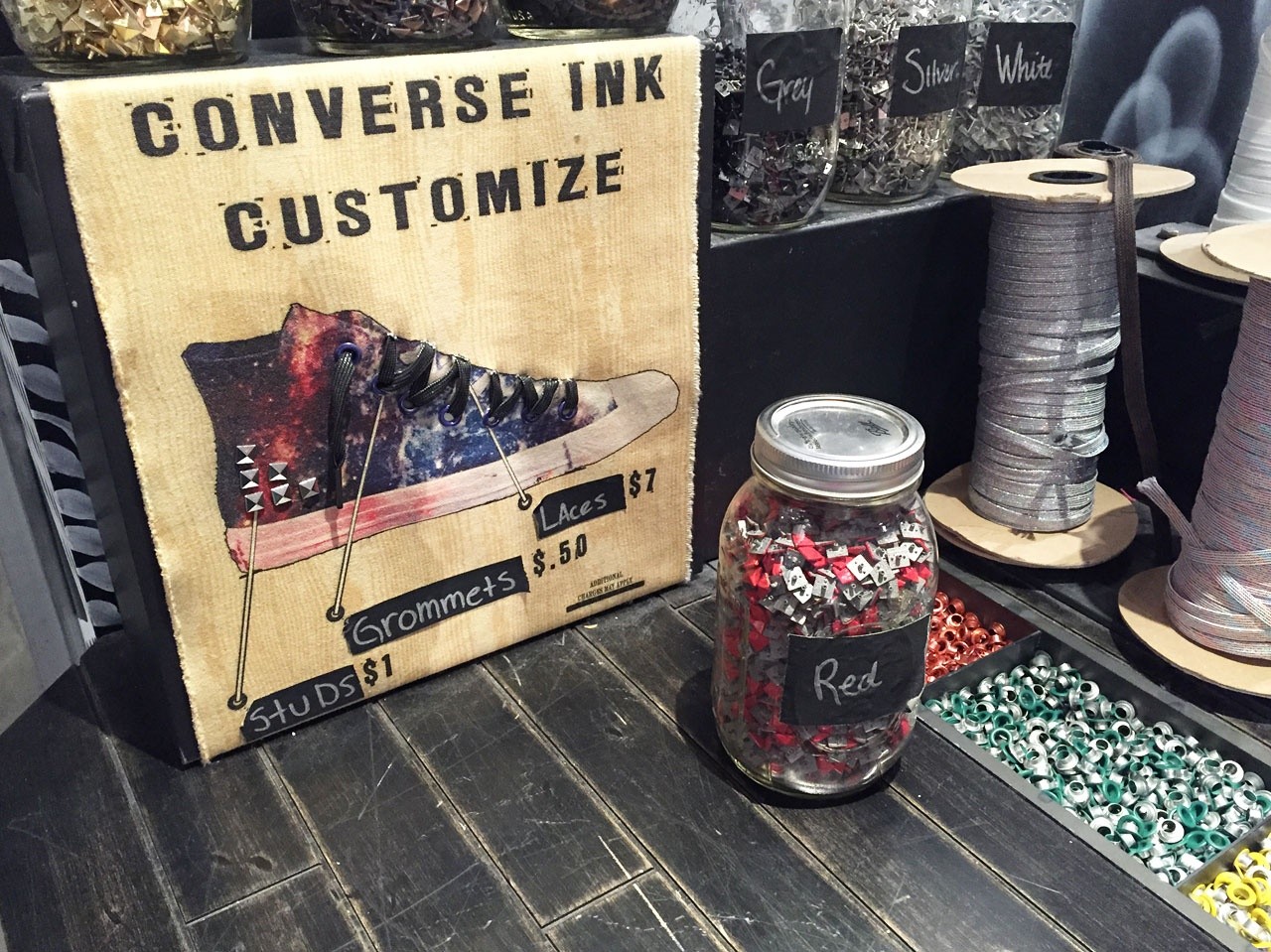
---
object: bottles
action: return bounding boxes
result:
[1,1,254,75]
[502,1,673,40]
[288,0,502,56]
[829,1,974,204]
[710,392,939,795]
[665,0,843,232]
[939,1,1086,178]
